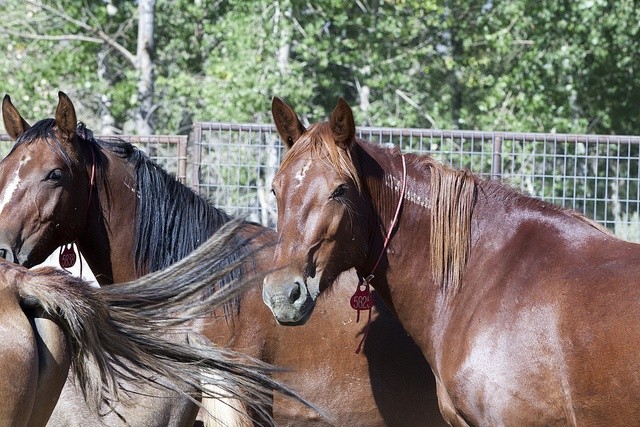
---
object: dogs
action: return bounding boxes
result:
[0,90,453,427]
[1,211,334,427]
[262,96,640,427]
[46,328,258,426]
